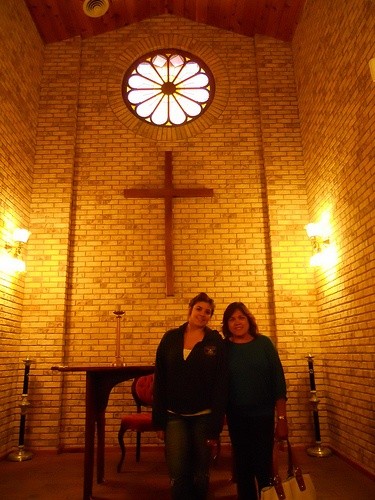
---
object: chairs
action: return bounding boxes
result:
[116,373,165,471]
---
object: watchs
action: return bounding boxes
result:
[276,416,286,419]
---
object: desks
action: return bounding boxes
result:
[50,366,156,500]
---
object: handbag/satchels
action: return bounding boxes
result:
[261,435,317,500]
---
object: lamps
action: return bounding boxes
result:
[5,230,29,271]
[306,222,332,266]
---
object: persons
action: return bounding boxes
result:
[149,292,224,500]
[221,301,287,500]
